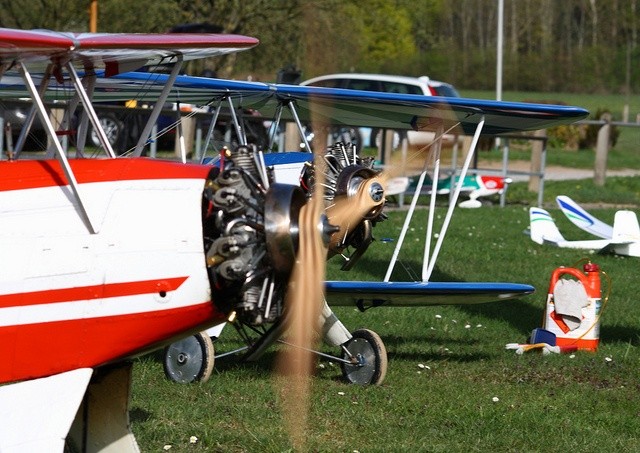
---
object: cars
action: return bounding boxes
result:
[1,81,216,149]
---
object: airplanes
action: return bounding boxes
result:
[0,20,341,453]
[2,58,591,390]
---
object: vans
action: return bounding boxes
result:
[262,71,473,150]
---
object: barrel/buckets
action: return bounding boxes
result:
[542,263,602,353]
